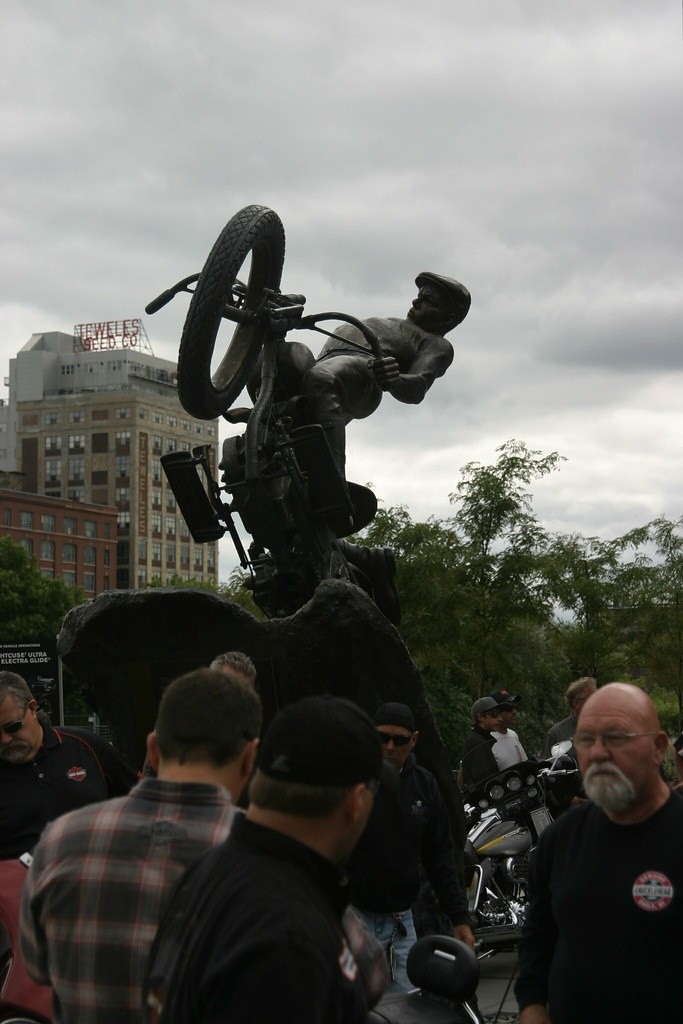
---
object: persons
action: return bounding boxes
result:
[216,272,471,534]
[517,682,682,1024]
[337,701,478,993]
[19,669,392,1024]
[139,694,388,1024]
[460,696,503,792]
[540,677,600,762]
[1,670,138,863]
[490,688,527,772]
[143,650,257,777]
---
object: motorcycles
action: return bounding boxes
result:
[446,735,577,961]
[144,203,388,618]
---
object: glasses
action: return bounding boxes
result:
[481,711,501,717]
[0,701,29,733]
[496,704,516,713]
[377,732,413,748]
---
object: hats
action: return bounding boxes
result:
[258,696,383,788]
[471,697,508,721]
[491,689,522,705]
[372,704,416,734]
